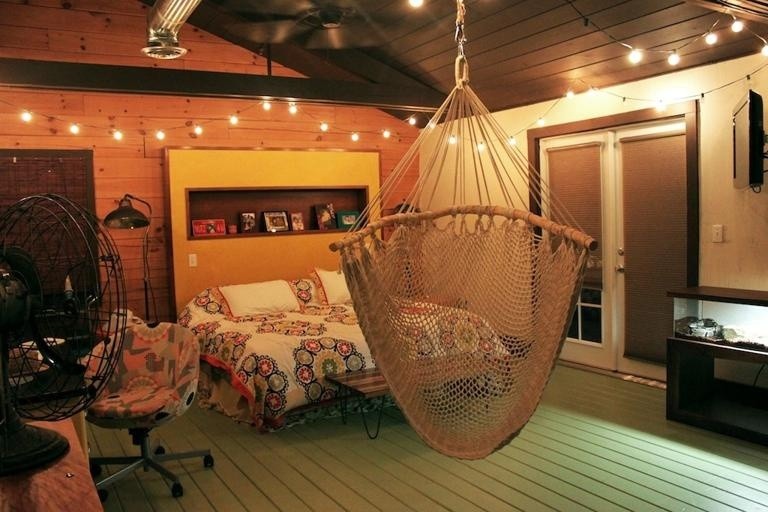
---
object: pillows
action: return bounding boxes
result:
[209,279,305,322]
[309,265,354,307]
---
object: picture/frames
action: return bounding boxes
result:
[192,219,226,237]
[239,208,257,233]
[261,211,293,232]
[337,210,360,230]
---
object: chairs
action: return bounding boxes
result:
[79,321,215,503]
[95,308,149,341]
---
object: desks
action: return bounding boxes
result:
[0,366,104,511]
[0,345,107,512]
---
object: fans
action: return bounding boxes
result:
[221,0,440,65]
[0,191,128,475]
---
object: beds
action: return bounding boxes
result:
[162,148,511,436]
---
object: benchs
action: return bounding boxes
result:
[322,349,500,439]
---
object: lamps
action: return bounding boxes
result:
[103,194,160,328]
[304,9,353,65]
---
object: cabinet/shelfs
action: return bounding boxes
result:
[666,286,767,446]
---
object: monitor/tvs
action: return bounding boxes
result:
[732,89,763,193]
